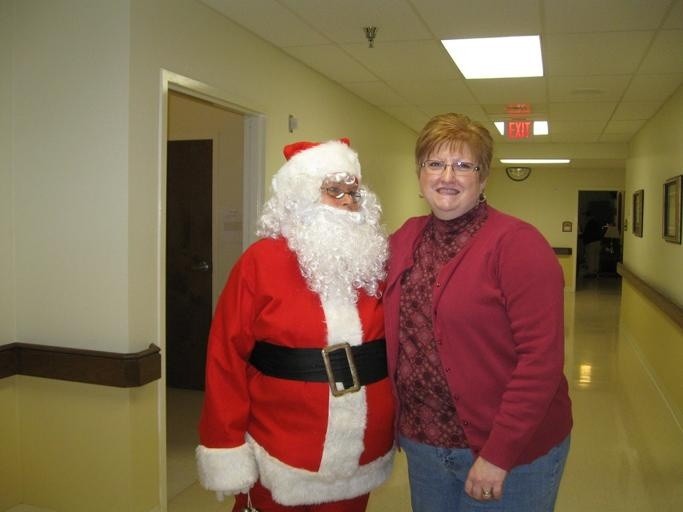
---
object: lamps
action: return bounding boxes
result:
[604,227,619,256]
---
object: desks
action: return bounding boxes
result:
[596,252,618,280]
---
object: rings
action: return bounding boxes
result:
[483,492,492,497]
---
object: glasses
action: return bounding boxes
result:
[422,160,480,174]
[322,187,361,202]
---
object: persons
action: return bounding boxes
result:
[194,138,397,512]
[384,112,572,512]
[578,210,604,272]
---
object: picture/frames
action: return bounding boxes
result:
[631,189,644,238]
[661,174,683,244]
